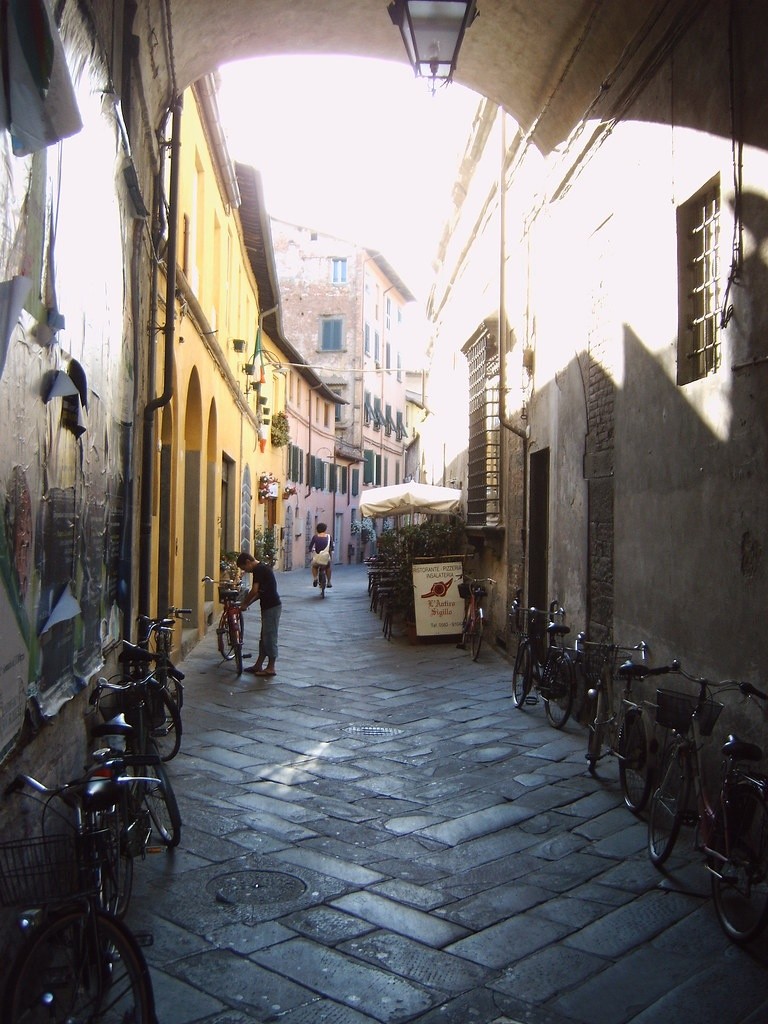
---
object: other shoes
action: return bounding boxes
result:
[313,580,317,586]
[327,585,332,588]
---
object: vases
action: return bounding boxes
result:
[282,492,289,499]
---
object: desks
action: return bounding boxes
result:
[363,556,402,641]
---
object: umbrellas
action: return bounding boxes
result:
[356,482,462,531]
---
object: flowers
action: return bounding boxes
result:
[258,472,280,501]
[284,484,299,496]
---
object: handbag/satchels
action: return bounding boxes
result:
[313,546,330,565]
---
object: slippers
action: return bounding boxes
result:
[256,671,276,676]
[244,667,256,672]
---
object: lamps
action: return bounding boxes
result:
[233,339,245,353]
[263,419,270,425]
[242,363,256,375]
[249,382,261,391]
[386,0,481,95]
[259,396,268,405]
[263,407,270,415]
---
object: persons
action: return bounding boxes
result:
[236,552,281,676]
[308,523,335,588]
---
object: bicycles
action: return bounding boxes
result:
[0,746,169,1023]
[574,631,660,814]
[103,605,192,762]
[510,588,577,729]
[309,549,335,599]
[202,576,252,676]
[82,667,186,921]
[640,660,768,944]
[455,574,498,662]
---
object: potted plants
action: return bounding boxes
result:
[379,521,462,646]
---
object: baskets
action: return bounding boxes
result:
[579,648,632,681]
[655,689,725,737]
[98,683,166,730]
[509,613,546,637]
[0,829,119,907]
[458,583,483,599]
[218,586,243,604]
